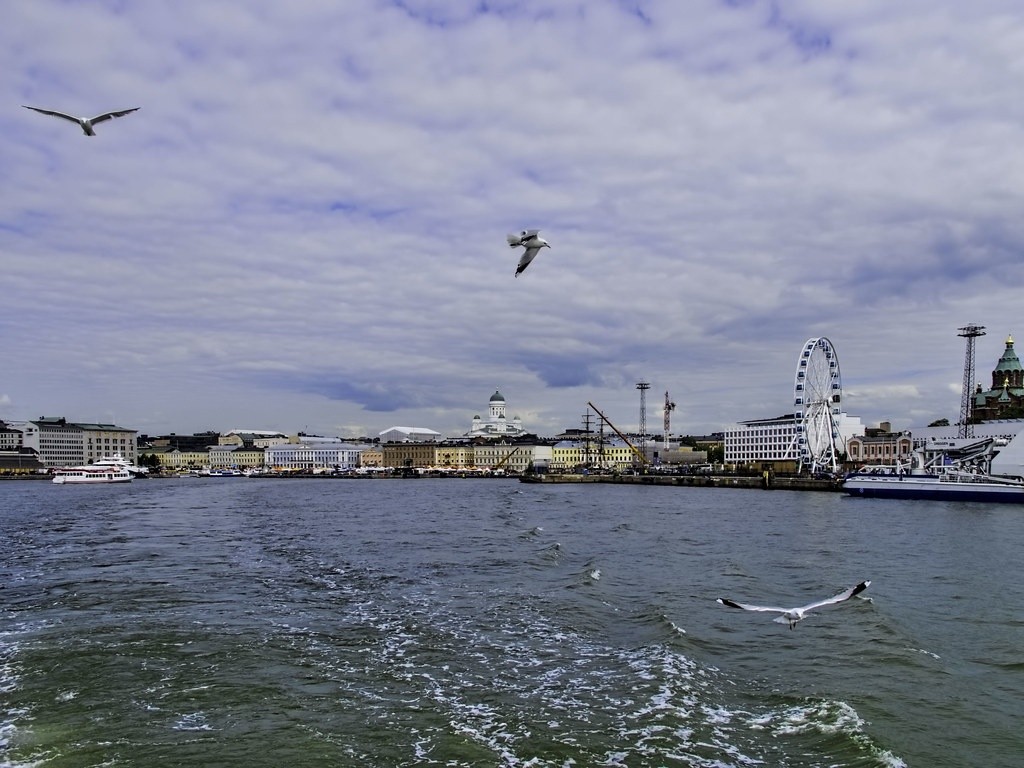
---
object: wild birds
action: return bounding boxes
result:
[20,102,145,137]
[504,227,553,279]
[714,578,874,632]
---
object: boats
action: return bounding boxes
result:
[519,459,554,482]
[842,437,1024,504]
[51,455,147,483]
